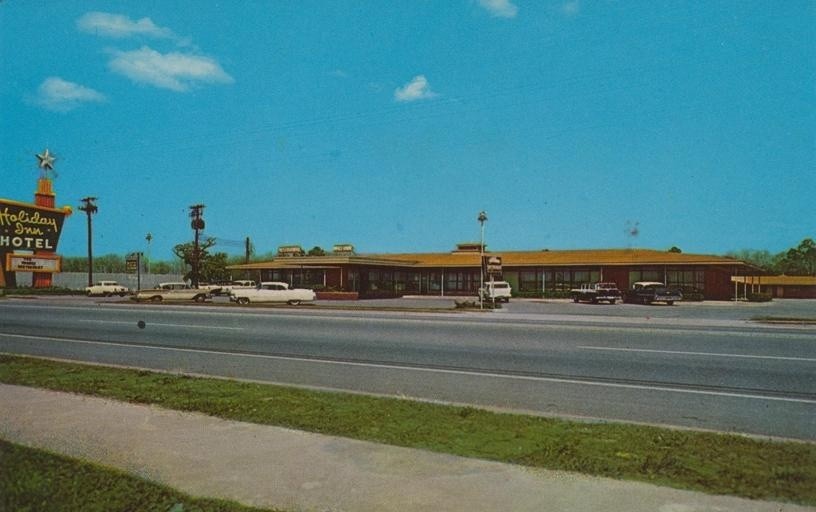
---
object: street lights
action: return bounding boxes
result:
[145,233,152,281]
[478,211,487,307]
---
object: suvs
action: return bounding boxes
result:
[479,281,512,303]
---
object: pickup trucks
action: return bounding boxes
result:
[136,281,211,302]
[571,282,683,307]
[230,282,313,306]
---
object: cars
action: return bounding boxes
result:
[85,280,128,296]
[200,281,255,295]
